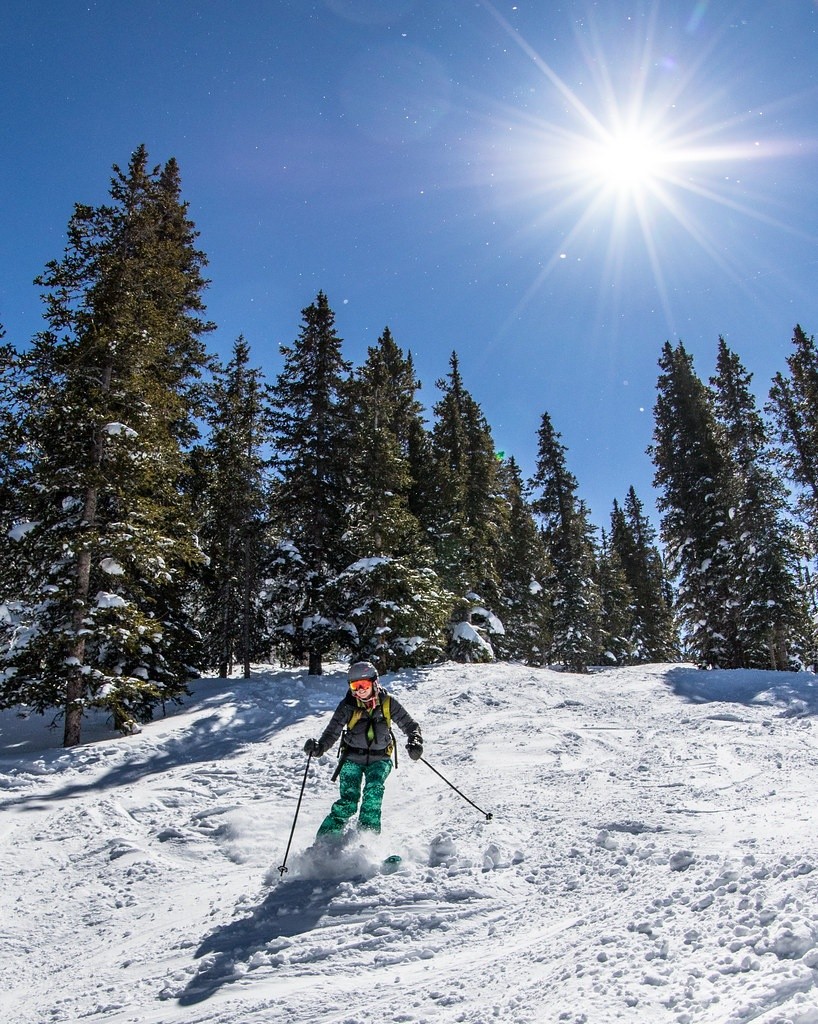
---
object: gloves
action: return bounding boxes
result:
[305,738,324,758]
[405,737,424,760]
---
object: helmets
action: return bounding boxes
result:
[347,662,378,691]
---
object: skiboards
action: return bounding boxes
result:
[304,846,402,873]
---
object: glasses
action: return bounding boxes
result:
[350,679,371,691]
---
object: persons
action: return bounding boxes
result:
[304,662,424,856]
